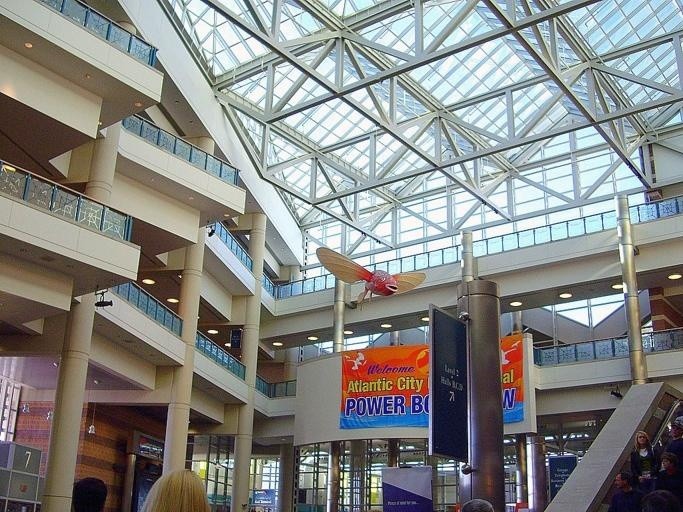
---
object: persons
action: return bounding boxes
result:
[608,416,683,512]
[140,469,212,512]
[73,477,107,512]
